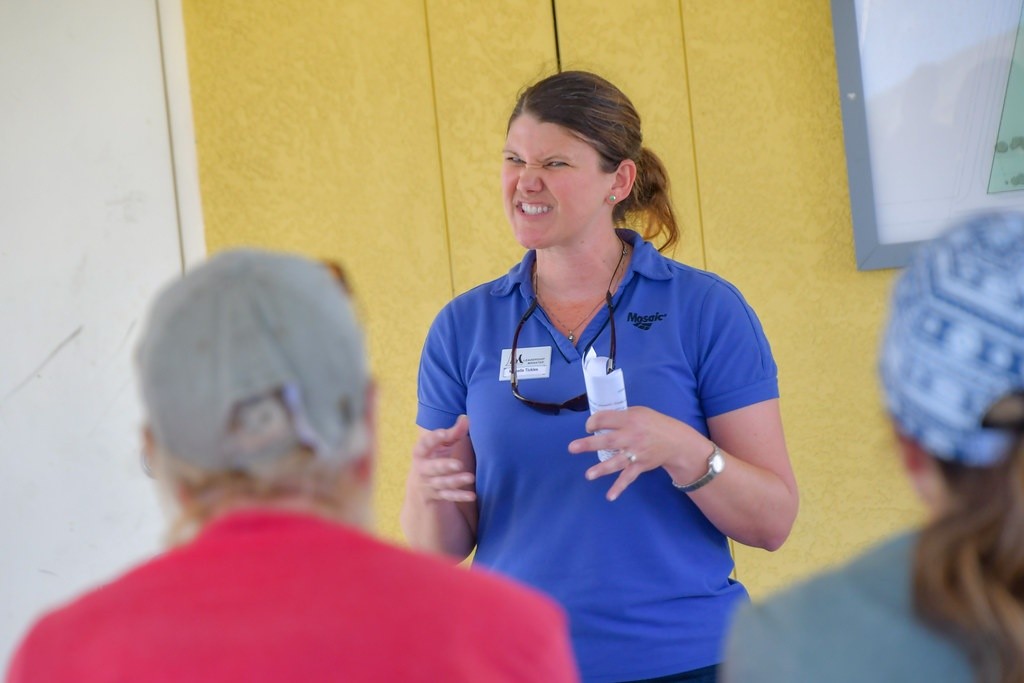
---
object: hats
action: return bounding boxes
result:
[877,210,1024,465]
[134,250,370,468]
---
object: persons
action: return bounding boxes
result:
[404,71,800,683]
[6,252,581,683]
[723,210,1023,683]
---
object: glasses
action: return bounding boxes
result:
[511,290,616,415]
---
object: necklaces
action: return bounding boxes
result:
[535,237,628,341]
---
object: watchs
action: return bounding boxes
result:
[673,441,724,491]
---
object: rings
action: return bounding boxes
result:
[623,450,636,464]
